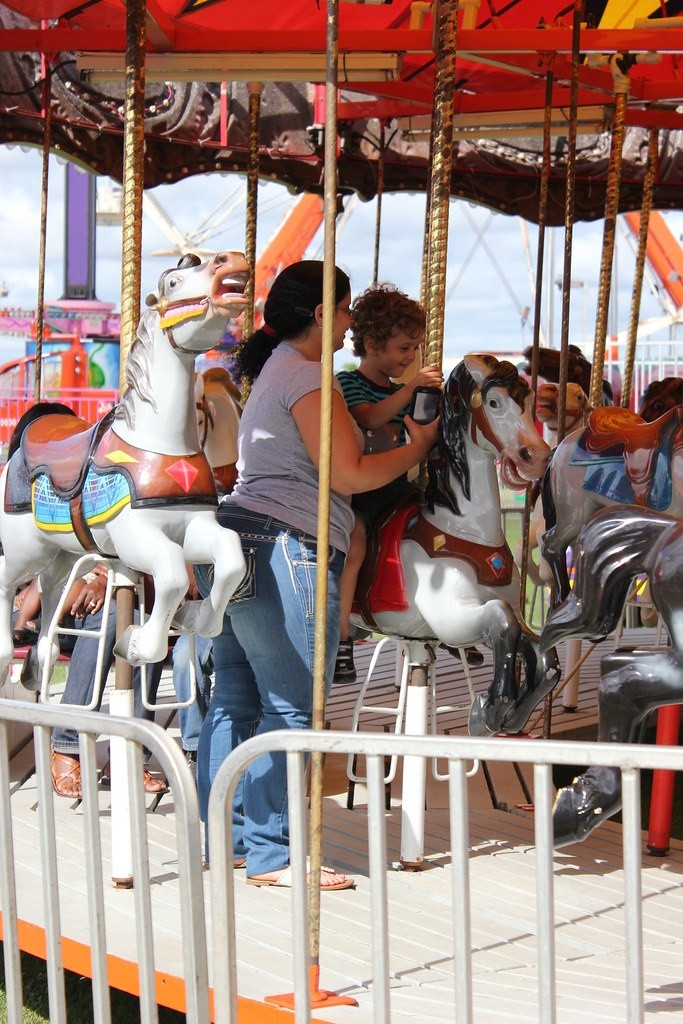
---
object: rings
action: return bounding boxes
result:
[90,602,96,607]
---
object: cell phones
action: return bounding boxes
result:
[410,387,441,426]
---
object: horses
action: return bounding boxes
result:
[0,252,250,693]
[536,382,591,446]
[531,404,683,617]
[348,351,562,737]
[522,344,683,424]
[537,505,683,850]
[196,368,243,496]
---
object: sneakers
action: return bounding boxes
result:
[331,636,357,684]
[439,643,483,666]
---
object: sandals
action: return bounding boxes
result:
[22,617,65,639]
[102,759,170,794]
[51,751,82,799]
[11,630,27,644]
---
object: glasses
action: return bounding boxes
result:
[313,303,355,320]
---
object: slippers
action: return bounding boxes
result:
[246,854,354,890]
[205,856,246,869]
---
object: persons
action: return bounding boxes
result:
[12,563,213,798]
[332,290,444,684]
[194,259,441,891]
